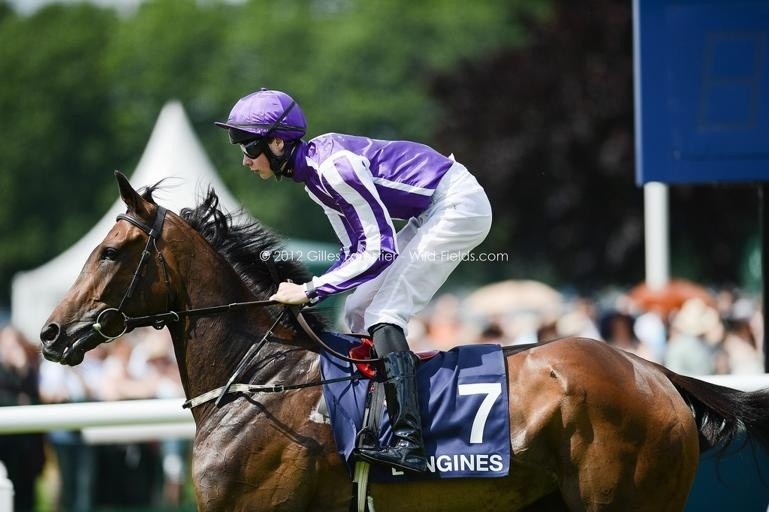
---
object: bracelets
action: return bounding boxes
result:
[307,281,320,306]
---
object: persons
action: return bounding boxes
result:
[215,88,500,475]
[0,273,769,511]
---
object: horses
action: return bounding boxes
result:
[36,169,769,512]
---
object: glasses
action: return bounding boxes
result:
[239,134,273,159]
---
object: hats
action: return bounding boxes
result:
[213,88,306,140]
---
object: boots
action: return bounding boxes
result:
[358,351,427,475]
